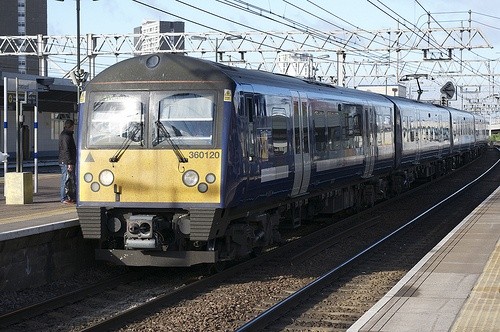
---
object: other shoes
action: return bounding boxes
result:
[70,199,76,204]
[61,201,72,205]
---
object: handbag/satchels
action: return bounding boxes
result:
[64,171,75,198]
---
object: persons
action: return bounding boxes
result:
[59,119,76,204]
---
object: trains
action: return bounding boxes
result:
[0,70,90,168]
[76,52,486,267]
